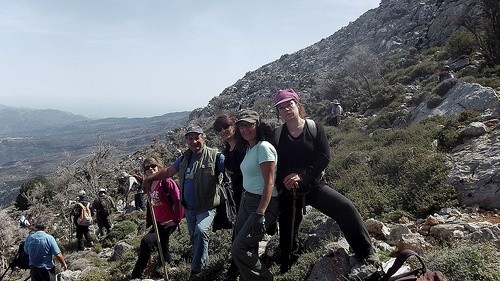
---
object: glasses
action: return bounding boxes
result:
[215,123,232,133]
[143,164,158,171]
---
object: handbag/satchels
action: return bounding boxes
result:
[211,186,236,232]
[372,249,445,281]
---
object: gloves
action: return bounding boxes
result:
[251,212,267,235]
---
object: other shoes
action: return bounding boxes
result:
[86,240,94,247]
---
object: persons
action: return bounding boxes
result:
[213,114,249,279]
[15,211,34,228]
[124,157,185,281]
[273,87,381,273]
[91,187,118,236]
[112,170,145,211]
[67,189,95,251]
[326,99,343,123]
[141,125,225,281]
[23,216,68,281]
[227,110,279,281]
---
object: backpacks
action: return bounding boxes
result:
[75,202,93,226]
[9,240,30,270]
[132,175,144,191]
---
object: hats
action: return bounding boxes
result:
[79,190,87,198]
[185,125,204,136]
[233,110,260,125]
[99,188,107,193]
[272,89,302,108]
